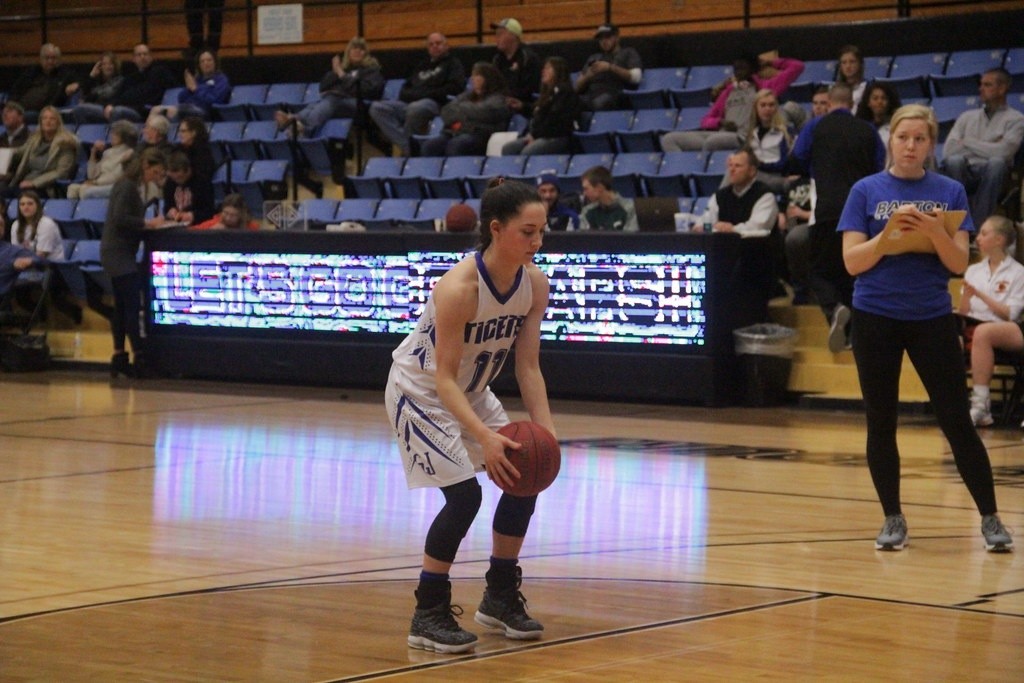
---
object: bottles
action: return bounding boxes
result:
[702,207,712,233]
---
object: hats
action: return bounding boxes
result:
[536,172,562,193]
[595,23,618,40]
[490,17,522,37]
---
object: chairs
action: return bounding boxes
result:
[1,47,1024,429]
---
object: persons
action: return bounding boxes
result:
[386,176,557,654]
[421,62,511,156]
[369,32,467,158]
[8,190,65,262]
[951,215,1024,425]
[99,146,168,378]
[835,103,1014,551]
[787,82,887,355]
[662,52,829,305]
[501,56,584,155]
[163,149,214,226]
[579,25,643,111]
[939,67,1024,229]
[195,192,260,231]
[0,203,39,291]
[185,0,226,52]
[0,44,231,200]
[534,165,640,233]
[489,18,542,117]
[840,45,902,145]
[276,35,385,139]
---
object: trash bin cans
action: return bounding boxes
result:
[733,323,799,409]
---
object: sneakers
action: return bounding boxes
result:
[875,514,909,552]
[981,517,1014,552]
[408,580,479,654]
[473,564,545,639]
[969,394,994,427]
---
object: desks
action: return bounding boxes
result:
[142,225,743,408]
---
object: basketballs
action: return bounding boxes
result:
[446,203,477,233]
[491,421,561,497]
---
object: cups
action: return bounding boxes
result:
[674,213,690,232]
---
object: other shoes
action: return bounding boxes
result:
[286,121,304,139]
[131,353,159,383]
[110,350,140,382]
[843,336,853,350]
[276,110,291,131]
[827,302,851,354]
